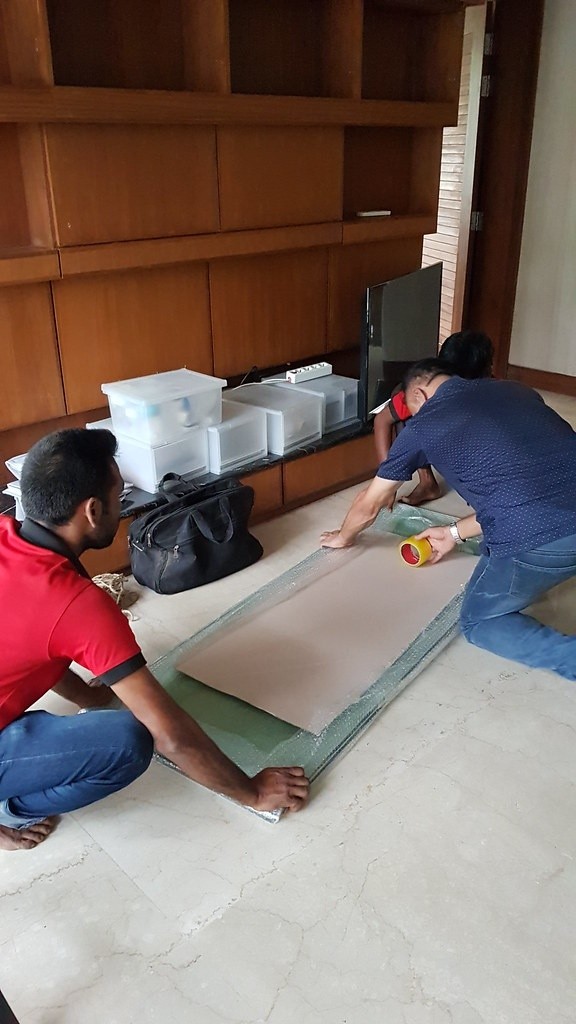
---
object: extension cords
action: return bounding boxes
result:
[286,362,332,384]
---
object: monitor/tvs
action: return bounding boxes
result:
[361,261,443,422]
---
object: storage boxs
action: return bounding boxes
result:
[100,367,228,447]
[86,414,210,496]
[220,382,325,459]
[205,400,267,476]
[262,370,360,432]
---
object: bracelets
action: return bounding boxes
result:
[449,523,466,545]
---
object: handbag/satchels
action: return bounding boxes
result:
[128,472,264,594]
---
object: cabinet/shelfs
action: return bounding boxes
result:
[0,0,471,434]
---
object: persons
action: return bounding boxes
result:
[0,428,309,852]
[318,357,576,682]
[373,329,495,506]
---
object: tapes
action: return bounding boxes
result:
[398,534,432,568]
